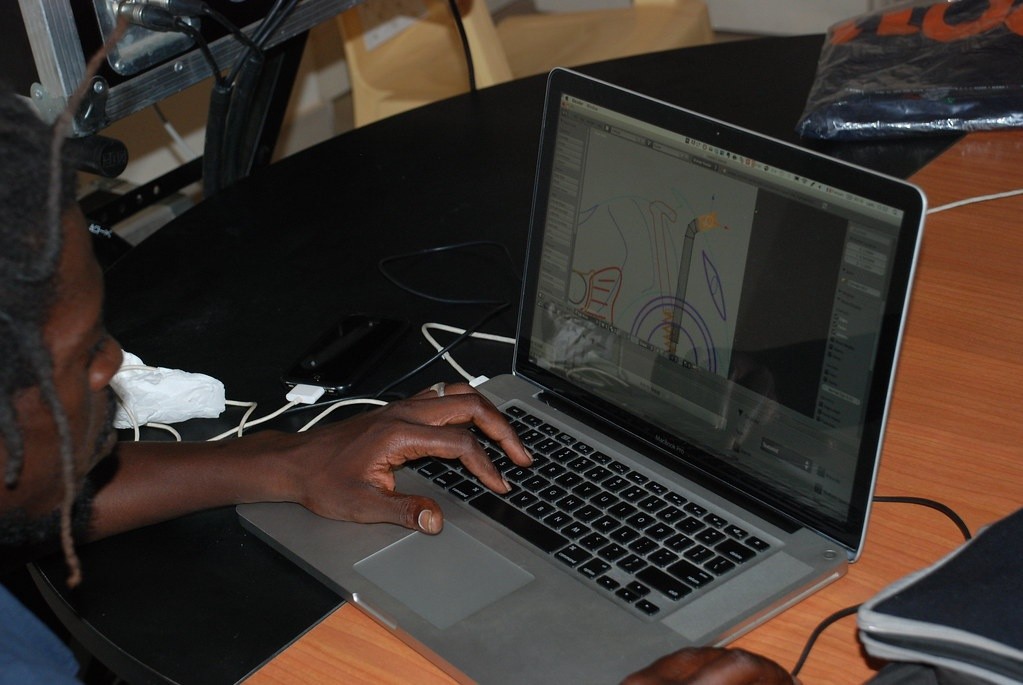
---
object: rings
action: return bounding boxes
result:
[430,382,445,397]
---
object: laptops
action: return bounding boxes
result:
[231,68,928,685]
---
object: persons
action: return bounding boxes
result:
[0,96,803,685]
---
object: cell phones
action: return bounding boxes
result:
[279,314,410,396]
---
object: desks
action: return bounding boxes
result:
[21,32,1023,685]
